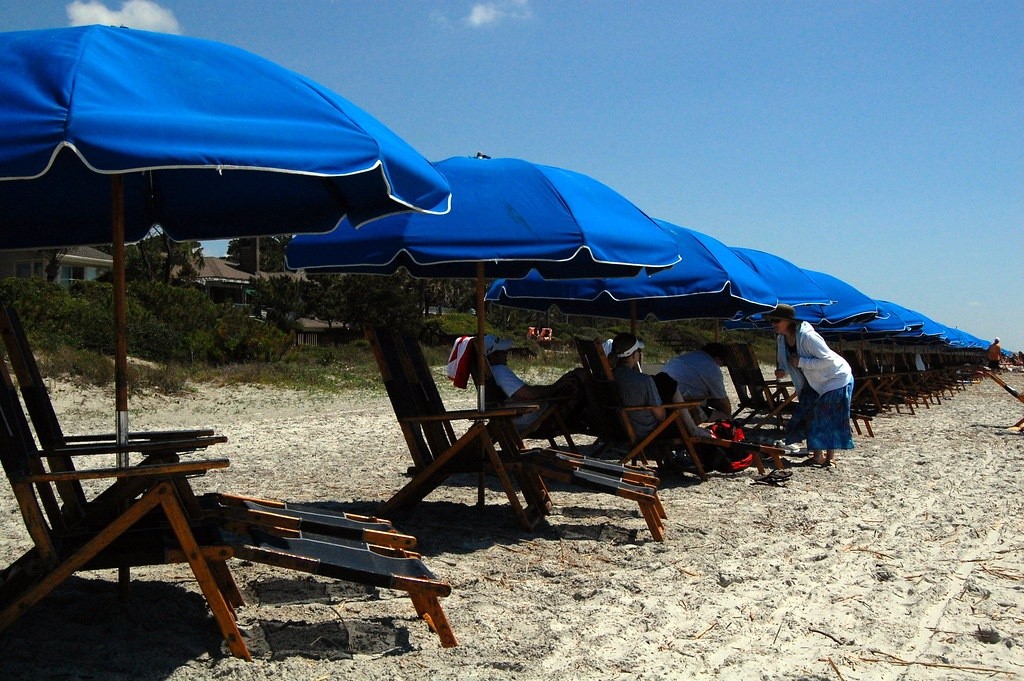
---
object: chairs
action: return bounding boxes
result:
[0,298,1024,663]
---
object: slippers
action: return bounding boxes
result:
[753,468,793,483]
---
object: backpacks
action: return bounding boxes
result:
[707,420,754,472]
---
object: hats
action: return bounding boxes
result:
[612,333,646,358]
[766,304,799,322]
[602,339,614,357]
[484,334,514,358]
[701,343,734,369]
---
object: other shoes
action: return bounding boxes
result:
[761,455,775,463]
[801,457,826,467]
[821,460,837,469]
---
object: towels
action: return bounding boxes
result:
[445,335,476,389]
[915,353,927,371]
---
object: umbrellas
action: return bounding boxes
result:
[285,153,685,504]
[0,22,453,593]
[728,245,1014,429]
[481,220,776,466]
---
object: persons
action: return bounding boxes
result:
[1011,352,1024,366]
[666,341,732,423]
[985,337,1001,374]
[612,333,714,438]
[484,331,551,432]
[760,304,855,468]
